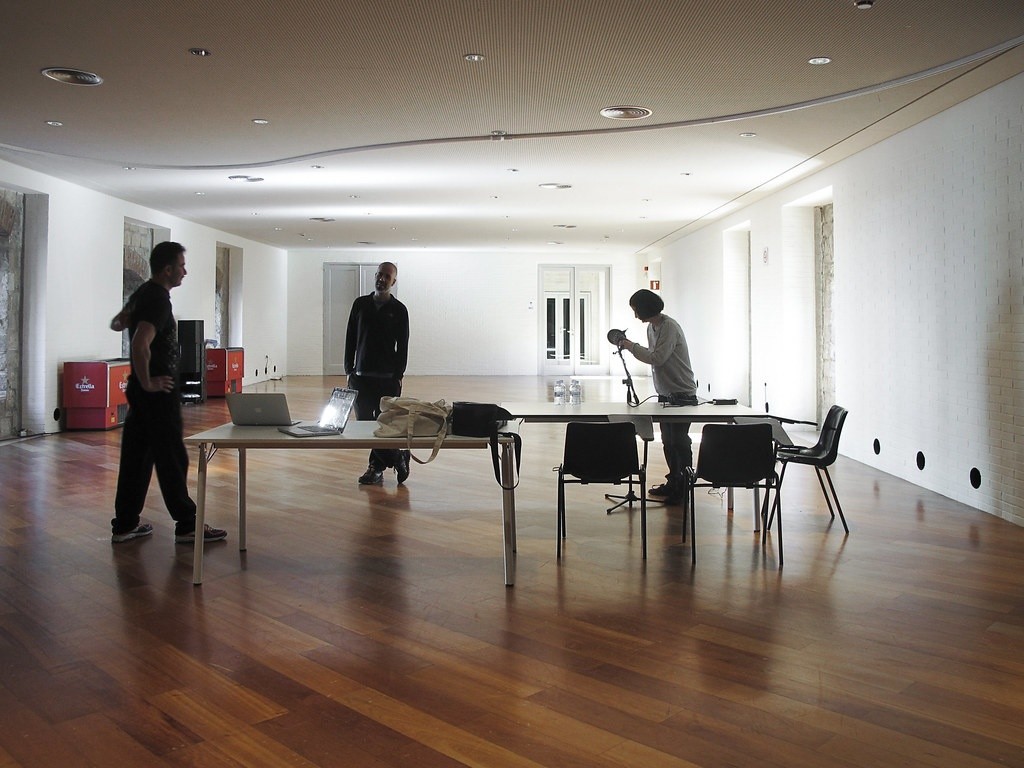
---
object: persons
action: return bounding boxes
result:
[343,262,410,486]
[614,289,699,496]
[110,240,228,543]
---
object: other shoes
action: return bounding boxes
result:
[649,474,673,496]
[665,486,686,504]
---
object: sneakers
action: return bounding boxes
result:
[175,524,227,543]
[112,523,153,541]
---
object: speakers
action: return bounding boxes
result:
[178,320,204,373]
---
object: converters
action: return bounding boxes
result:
[713,398,738,405]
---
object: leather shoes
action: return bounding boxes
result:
[394,450,410,482]
[359,469,384,484]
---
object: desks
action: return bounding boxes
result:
[184,418,525,587]
[501,402,771,533]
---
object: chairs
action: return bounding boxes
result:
[681,423,783,568]
[761,404,850,534]
[557,420,647,559]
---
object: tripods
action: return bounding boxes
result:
[605,342,665,513]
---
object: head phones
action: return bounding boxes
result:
[668,393,698,406]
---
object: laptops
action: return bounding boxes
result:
[225,392,303,426]
[279,387,359,438]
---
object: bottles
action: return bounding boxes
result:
[554,381,563,404]
[572,381,582,405]
[568,379,577,403]
[559,379,566,404]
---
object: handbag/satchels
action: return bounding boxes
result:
[452,401,521,490]
[373,395,448,464]
